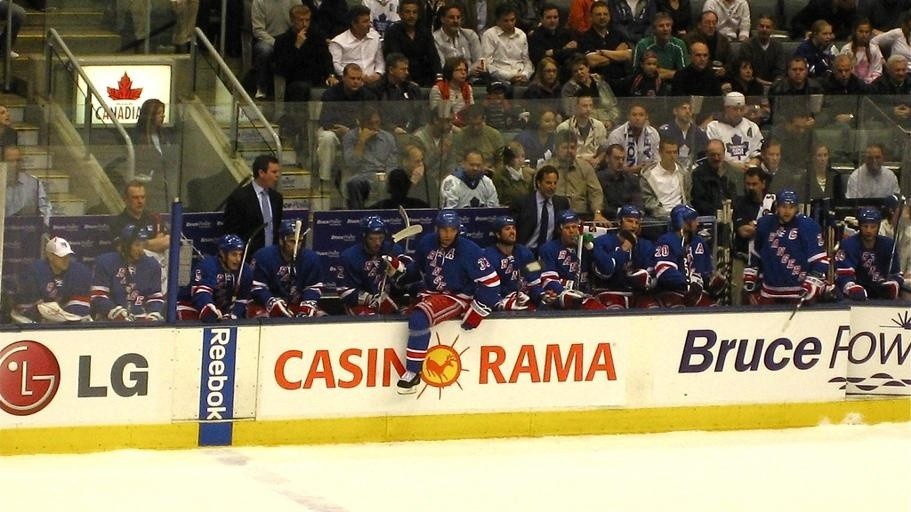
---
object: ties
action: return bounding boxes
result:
[536,200,548,246]
[260,190,272,244]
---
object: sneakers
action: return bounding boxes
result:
[396,369,420,395]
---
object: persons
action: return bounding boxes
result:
[725,61,766,122]
[304,0,346,41]
[113,181,171,255]
[543,212,613,308]
[510,1,545,31]
[382,4,441,81]
[464,0,499,33]
[834,208,903,300]
[847,144,902,196]
[253,219,327,317]
[343,107,406,209]
[431,7,486,80]
[598,202,662,308]
[9,237,91,323]
[513,109,559,164]
[794,20,840,77]
[659,99,708,161]
[224,154,284,259]
[510,166,571,248]
[840,21,883,83]
[315,64,375,190]
[767,111,822,161]
[453,105,505,171]
[703,0,750,40]
[756,141,792,193]
[641,141,693,217]
[253,0,303,97]
[338,216,411,314]
[477,83,513,128]
[372,53,424,132]
[396,211,501,394]
[869,56,911,127]
[872,11,911,74]
[530,6,584,60]
[438,151,500,207]
[562,54,620,129]
[744,189,829,304]
[276,5,339,128]
[735,16,787,82]
[176,231,255,321]
[0,106,18,147]
[328,7,385,87]
[566,0,598,30]
[730,169,775,306]
[390,146,439,210]
[881,197,911,289]
[364,0,400,33]
[682,13,734,78]
[480,7,535,93]
[634,13,690,84]
[631,51,664,104]
[414,106,461,176]
[0,1,24,58]
[430,57,474,116]
[824,55,872,124]
[769,59,828,129]
[526,59,563,119]
[605,105,661,167]
[368,170,430,210]
[654,204,726,306]
[0,145,51,223]
[672,43,722,128]
[706,93,764,169]
[484,216,555,310]
[598,146,645,216]
[584,3,631,78]
[92,225,166,323]
[132,0,197,56]
[608,0,648,28]
[802,144,842,200]
[535,132,613,227]
[132,100,179,211]
[693,140,745,215]
[657,0,691,35]
[556,88,606,169]
[490,143,535,205]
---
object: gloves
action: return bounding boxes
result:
[296,302,318,319]
[267,297,294,319]
[616,228,638,245]
[498,295,527,313]
[377,254,404,279]
[882,278,899,299]
[135,311,163,323]
[800,273,823,304]
[844,281,867,301]
[684,271,704,304]
[506,289,530,304]
[742,266,758,291]
[627,266,653,292]
[558,286,584,309]
[461,300,486,330]
[112,306,132,321]
[198,300,220,320]
[708,269,727,296]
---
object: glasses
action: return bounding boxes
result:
[450,66,468,73]
[540,68,559,74]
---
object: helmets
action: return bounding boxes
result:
[494,215,517,231]
[434,209,460,245]
[359,215,388,255]
[121,224,148,241]
[557,212,582,229]
[670,205,696,231]
[278,216,302,236]
[775,188,801,213]
[857,206,880,226]
[616,206,641,221]
[216,234,243,252]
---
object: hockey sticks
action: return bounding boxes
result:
[285,220,302,311]
[228,221,269,319]
[886,192,906,281]
[619,228,638,260]
[377,223,423,314]
[680,227,691,292]
[782,220,841,332]
[574,213,595,290]
[398,203,410,255]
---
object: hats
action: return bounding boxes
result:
[727,92,746,108]
[487,81,506,92]
[45,237,76,258]
